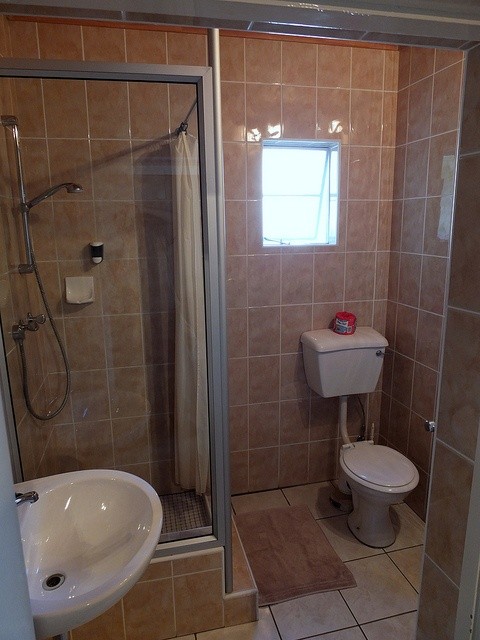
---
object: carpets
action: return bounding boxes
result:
[233,504,358,608]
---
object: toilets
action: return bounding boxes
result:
[301,326,421,548]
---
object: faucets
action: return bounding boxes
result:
[15,490,39,507]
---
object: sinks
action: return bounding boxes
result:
[15,469,165,638]
